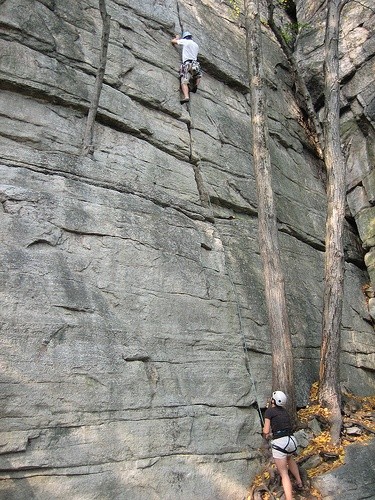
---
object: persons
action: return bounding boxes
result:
[263,390,304,500]
[171,32,201,103]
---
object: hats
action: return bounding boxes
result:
[183,31,192,38]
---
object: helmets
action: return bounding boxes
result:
[272,391,287,407]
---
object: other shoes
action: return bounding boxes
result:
[293,482,304,491]
[192,85,197,93]
[181,98,190,104]
